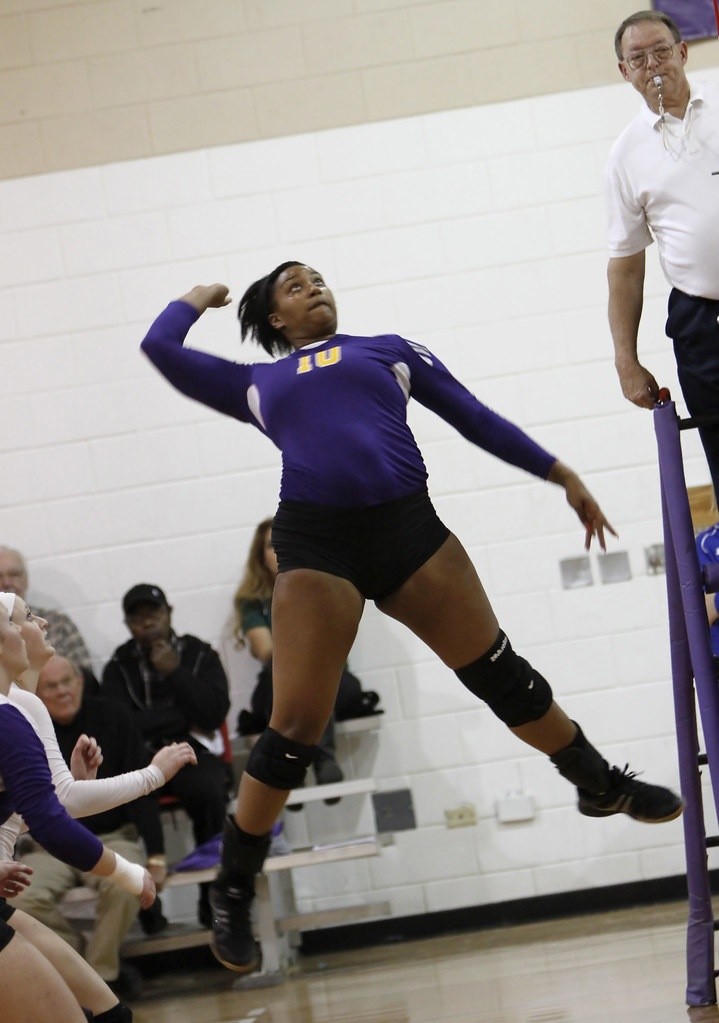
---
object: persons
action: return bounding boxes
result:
[0,520,348,1023]
[610,11,719,504]
[137,260,685,974]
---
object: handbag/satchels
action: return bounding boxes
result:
[334,668,378,721]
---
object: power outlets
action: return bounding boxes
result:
[442,804,477,829]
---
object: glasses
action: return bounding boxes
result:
[126,606,165,624]
[623,41,679,71]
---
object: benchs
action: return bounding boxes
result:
[55,718,389,988]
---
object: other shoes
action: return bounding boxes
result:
[311,754,343,805]
[284,782,305,812]
[135,893,167,935]
[198,878,212,929]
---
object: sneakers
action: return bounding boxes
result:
[578,761,684,823]
[208,876,255,970]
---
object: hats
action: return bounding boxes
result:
[124,585,165,615]
